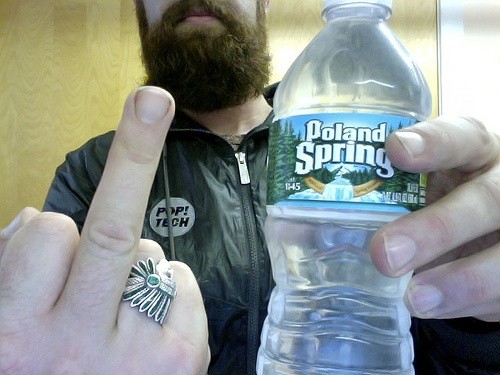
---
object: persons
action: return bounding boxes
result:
[0,0,500,375]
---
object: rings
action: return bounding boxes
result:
[121,257,177,326]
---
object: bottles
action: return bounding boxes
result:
[255,1,433,375]
